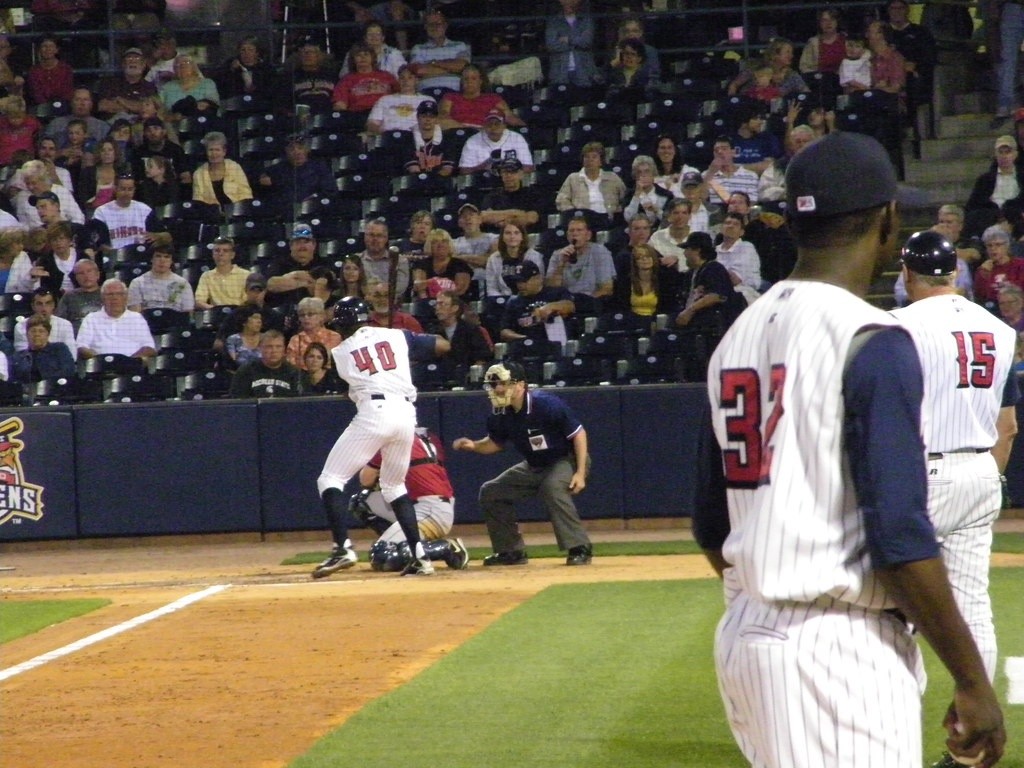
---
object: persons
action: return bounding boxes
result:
[692,131,1007,768]
[452,360,592,566]
[312,296,451,579]
[0,0,1024,407]
[885,230,1018,768]
[348,427,468,577]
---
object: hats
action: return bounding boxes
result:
[16,139,536,267]
[397,63,418,75]
[682,170,705,187]
[675,231,713,248]
[483,108,506,123]
[417,100,439,116]
[123,48,143,58]
[350,44,372,57]
[992,134,1019,150]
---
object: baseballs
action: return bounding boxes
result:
[947,722,985,765]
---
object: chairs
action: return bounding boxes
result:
[0,61,938,406]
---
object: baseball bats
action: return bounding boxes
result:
[388,245,399,329]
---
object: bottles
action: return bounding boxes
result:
[568,238,578,264]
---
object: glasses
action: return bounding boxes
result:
[286,136,304,145]
[291,228,311,235]
[116,173,134,179]
[214,236,235,248]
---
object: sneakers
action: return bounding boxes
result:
[313,537,357,578]
[397,555,435,576]
[444,537,470,570]
[566,544,595,564]
[483,548,529,566]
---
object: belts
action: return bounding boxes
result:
[412,495,451,505]
[928,448,990,460]
[369,392,412,403]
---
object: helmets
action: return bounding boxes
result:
[326,295,371,324]
[784,131,897,218]
[482,362,527,384]
[895,229,958,275]
